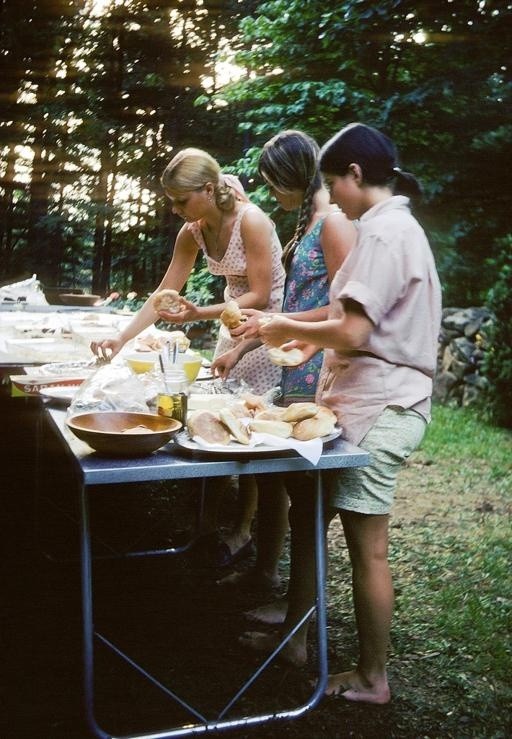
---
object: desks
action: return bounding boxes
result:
[22,347,374,737]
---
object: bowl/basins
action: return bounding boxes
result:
[9,288,205,456]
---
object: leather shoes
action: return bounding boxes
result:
[211,535,252,566]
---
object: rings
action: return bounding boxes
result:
[239,334,245,341]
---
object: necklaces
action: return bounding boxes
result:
[204,208,226,254]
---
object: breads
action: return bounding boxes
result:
[186,394,337,445]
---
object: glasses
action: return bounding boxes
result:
[323,174,340,193]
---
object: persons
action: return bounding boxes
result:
[86,145,285,572]
[227,121,444,708]
[201,128,354,630]
